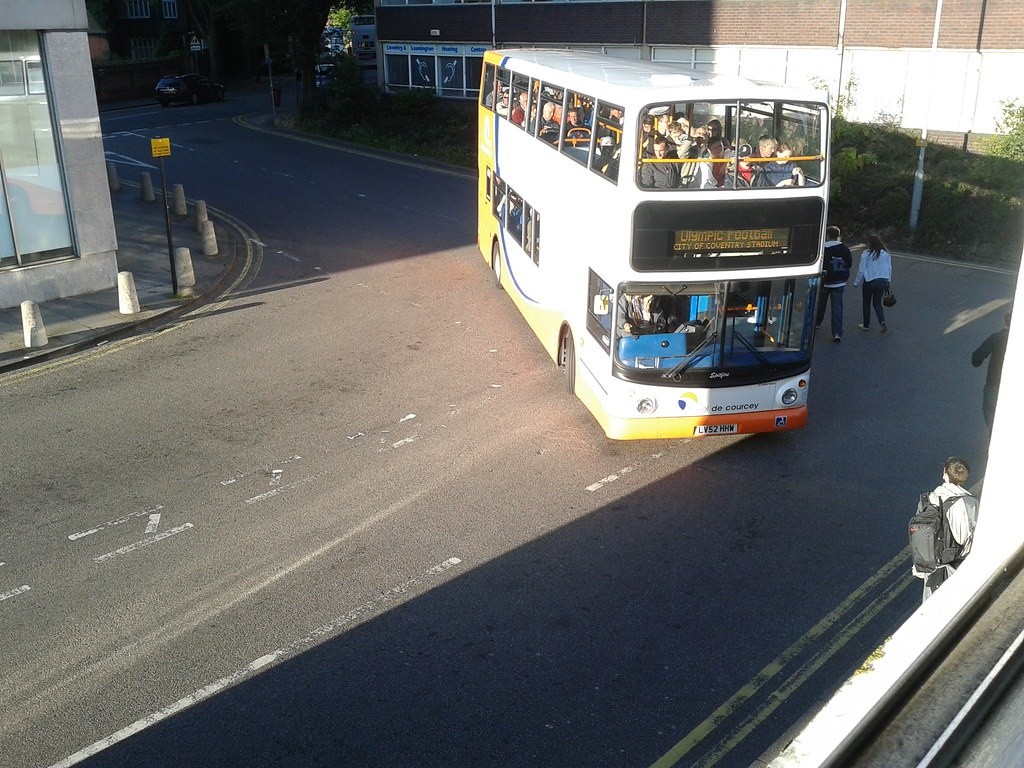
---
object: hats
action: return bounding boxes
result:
[600,136,615,147]
[643,115,653,125]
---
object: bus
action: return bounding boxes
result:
[349,13,377,70]
[475,44,833,443]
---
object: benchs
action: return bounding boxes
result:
[539,127,601,147]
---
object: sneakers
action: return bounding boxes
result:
[833,333,840,342]
[815,325,820,329]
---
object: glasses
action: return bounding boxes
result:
[503,96,509,99]
[707,127,712,131]
[522,99,527,101]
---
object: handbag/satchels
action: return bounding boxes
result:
[883,286,897,308]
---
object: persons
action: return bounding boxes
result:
[909,458,978,605]
[617,293,687,334]
[485,79,624,179]
[853,235,892,334]
[972,313,1012,443]
[640,112,805,188]
[815,226,853,343]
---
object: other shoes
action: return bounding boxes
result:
[858,323,870,332]
[880,321,888,334]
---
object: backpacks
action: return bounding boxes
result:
[908,492,969,573]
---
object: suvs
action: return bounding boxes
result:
[154,71,225,107]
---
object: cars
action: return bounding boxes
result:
[294,28,344,90]
